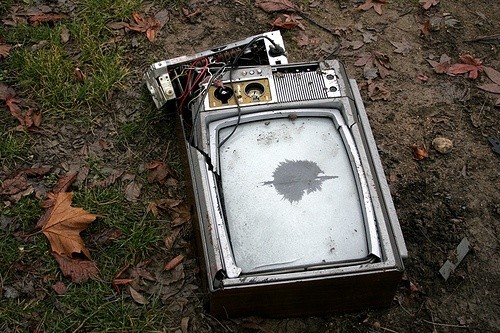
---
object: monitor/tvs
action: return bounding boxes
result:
[145,29,408,320]
